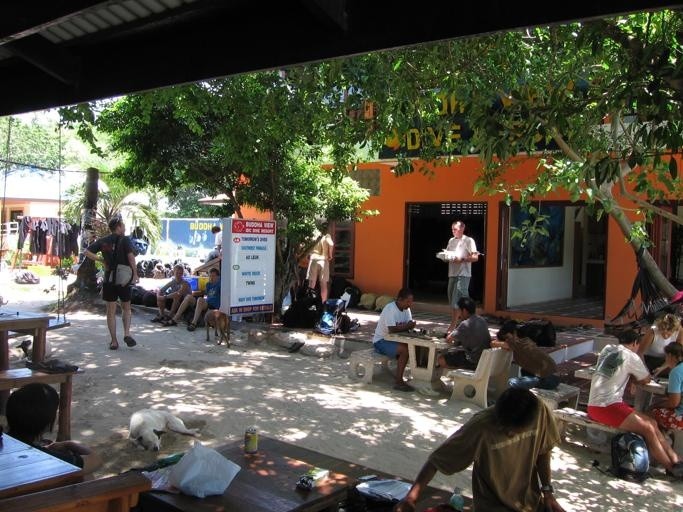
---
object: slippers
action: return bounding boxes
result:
[109,338,120,351]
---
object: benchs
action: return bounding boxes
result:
[548,337,599,385]
[552,407,629,455]
[352,347,390,384]
[0,366,86,440]
[447,346,514,408]
[0,472,153,512]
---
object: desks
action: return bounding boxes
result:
[140,434,379,512]
[574,364,669,413]
[0,305,56,370]
[0,431,83,501]
[386,327,501,389]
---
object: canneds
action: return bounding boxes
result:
[244,428,258,454]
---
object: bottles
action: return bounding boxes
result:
[449,486,465,512]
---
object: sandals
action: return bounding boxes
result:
[150,313,198,332]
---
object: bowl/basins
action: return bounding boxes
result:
[421,329,433,336]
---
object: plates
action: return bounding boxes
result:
[355,479,415,504]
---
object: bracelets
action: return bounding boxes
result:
[462,258,464,261]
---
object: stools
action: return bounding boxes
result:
[529,384,580,414]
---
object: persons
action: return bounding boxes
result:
[150,225,334,331]
[373,287,416,392]
[392,386,568,512]
[445,220,479,335]
[586,329,683,480]
[5,383,91,469]
[637,313,683,379]
[438,297,491,371]
[82,217,140,350]
[490,325,560,391]
[647,342,683,439]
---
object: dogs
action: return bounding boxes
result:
[204,309,231,348]
[126,407,201,453]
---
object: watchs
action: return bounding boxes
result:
[542,485,554,492]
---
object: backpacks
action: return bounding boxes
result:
[609,431,650,483]
[281,287,346,334]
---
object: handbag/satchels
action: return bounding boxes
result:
[106,264,135,289]
[519,318,557,348]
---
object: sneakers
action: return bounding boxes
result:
[665,460,683,480]
[122,335,137,347]
[393,380,414,392]
